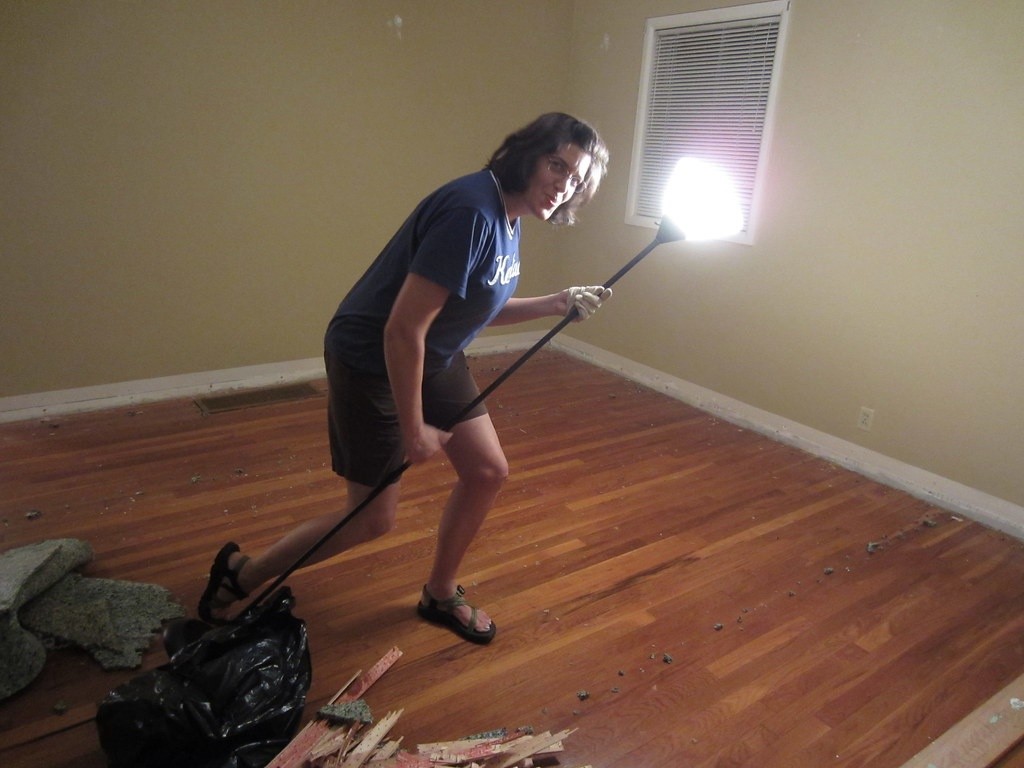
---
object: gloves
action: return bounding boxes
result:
[564,286,612,323]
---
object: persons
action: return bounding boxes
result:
[198,112,612,644]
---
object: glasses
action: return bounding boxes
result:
[545,153,587,193]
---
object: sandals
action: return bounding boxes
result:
[416,584,496,642]
[197,541,249,617]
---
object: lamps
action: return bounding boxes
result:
[160,153,747,659]
